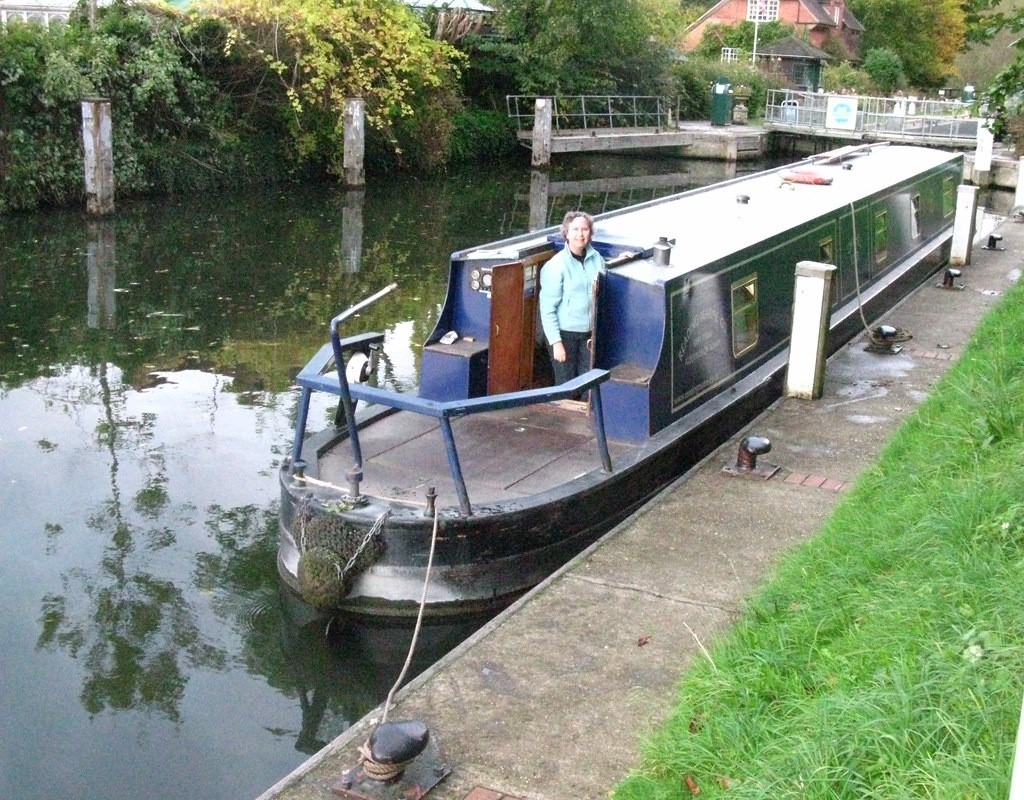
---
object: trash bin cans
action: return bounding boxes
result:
[733,105,750,125]
[710,82,734,126]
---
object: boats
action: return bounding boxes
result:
[276,142,1009,629]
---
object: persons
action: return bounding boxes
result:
[539,210,607,401]
